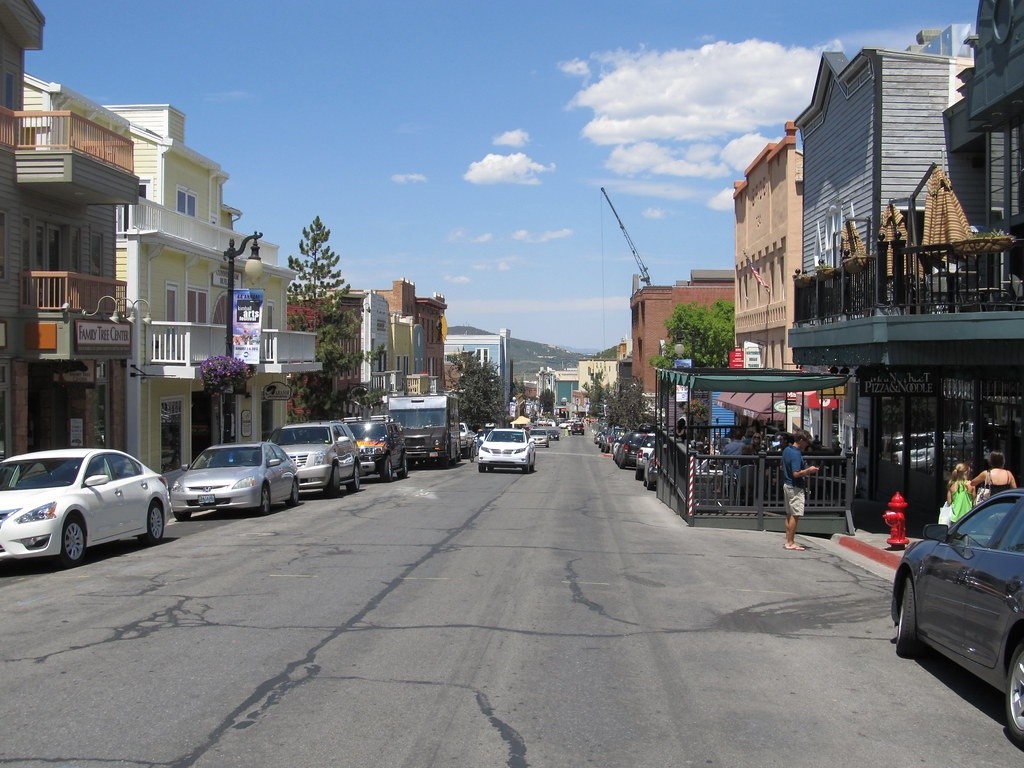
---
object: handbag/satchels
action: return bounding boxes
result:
[973,470,990,507]
[939,501,952,529]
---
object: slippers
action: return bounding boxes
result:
[784,544,806,551]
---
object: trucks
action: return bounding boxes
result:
[382,393,460,469]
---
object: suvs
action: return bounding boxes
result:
[341,415,408,482]
[266,421,363,497]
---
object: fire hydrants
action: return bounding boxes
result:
[882,492,910,550]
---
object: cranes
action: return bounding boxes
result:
[600,187,652,287]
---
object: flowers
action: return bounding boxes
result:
[200,354,251,397]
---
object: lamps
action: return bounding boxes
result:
[82,295,120,325]
[101,297,136,324]
[120,298,153,325]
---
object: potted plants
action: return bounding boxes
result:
[815,265,834,277]
[844,251,869,273]
[794,274,812,288]
[954,228,1016,253]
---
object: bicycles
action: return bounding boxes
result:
[467,436,481,463]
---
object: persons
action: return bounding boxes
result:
[716,423,794,505]
[947,463,976,532]
[968,450,1017,499]
[779,430,819,552]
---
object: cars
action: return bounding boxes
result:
[892,488,1024,747]
[594,424,659,491]
[529,418,585,448]
[170,442,300,522]
[0,448,170,568]
[460,422,474,459]
[882,420,1013,473]
[478,428,536,473]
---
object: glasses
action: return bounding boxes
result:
[754,436,760,439]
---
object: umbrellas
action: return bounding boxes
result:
[918,168,973,280]
[876,202,916,277]
[839,219,866,273]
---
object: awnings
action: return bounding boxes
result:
[796,391,839,409]
[714,392,785,422]
